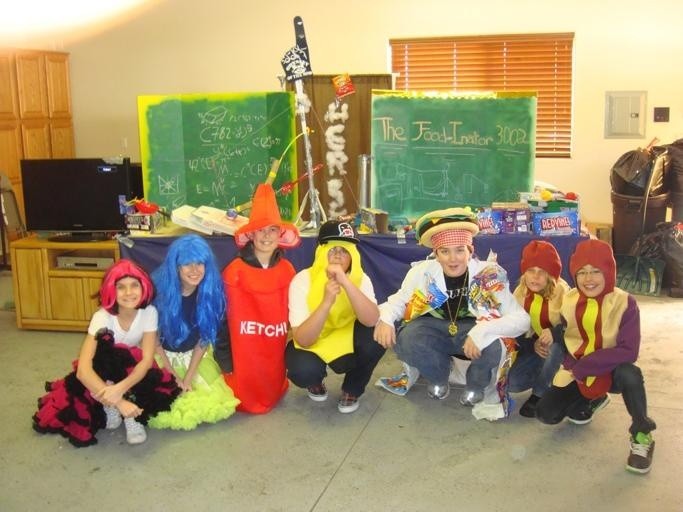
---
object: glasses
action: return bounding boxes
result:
[574,270,599,278]
[329,248,347,257]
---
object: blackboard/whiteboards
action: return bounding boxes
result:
[371,87,538,223]
[137,91,299,224]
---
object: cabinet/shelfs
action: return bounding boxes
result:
[0,48,80,247]
[284,73,393,218]
[9,233,124,335]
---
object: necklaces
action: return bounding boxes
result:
[440,271,467,335]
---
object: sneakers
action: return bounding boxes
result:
[459,388,484,406]
[519,395,540,417]
[104,380,122,430]
[625,439,655,473]
[338,393,359,414]
[125,414,147,444]
[307,382,330,400]
[426,382,450,400]
[566,392,611,424]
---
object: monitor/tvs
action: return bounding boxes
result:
[21,157,131,242]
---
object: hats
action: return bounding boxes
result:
[235,184,302,247]
[414,207,482,253]
[317,220,360,244]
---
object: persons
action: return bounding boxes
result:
[220,223,297,415]
[74,260,158,446]
[373,209,529,407]
[536,239,657,474]
[284,221,387,414]
[505,241,571,418]
[148,233,240,431]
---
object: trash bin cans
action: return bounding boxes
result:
[671,192,683,223]
[611,191,671,268]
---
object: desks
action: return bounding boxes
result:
[120,216,589,345]
